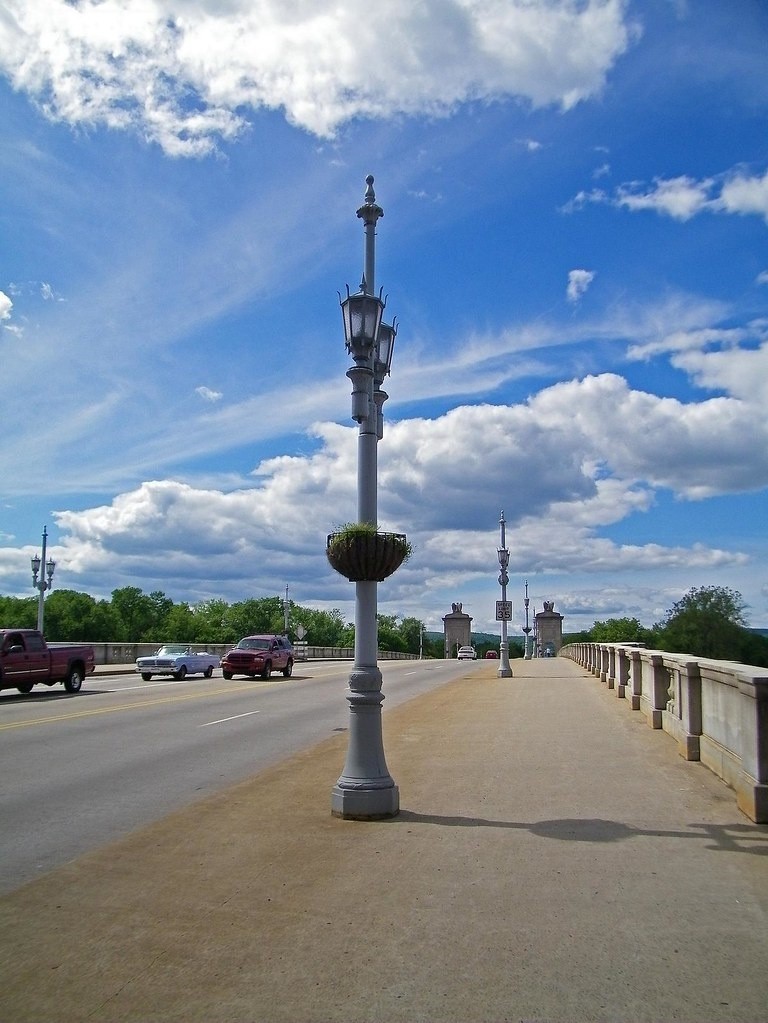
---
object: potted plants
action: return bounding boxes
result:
[327,520,401,579]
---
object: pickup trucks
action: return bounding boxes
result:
[0,629,96,693]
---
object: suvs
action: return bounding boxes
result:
[222,633,294,680]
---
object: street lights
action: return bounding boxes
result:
[531,606,538,658]
[283,584,290,637]
[522,580,531,659]
[497,509,512,677]
[31,524,55,635]
[331,175,401,822]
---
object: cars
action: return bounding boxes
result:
[135,646,220,682]
[486,650,497,659]
[458,646,477,661]
